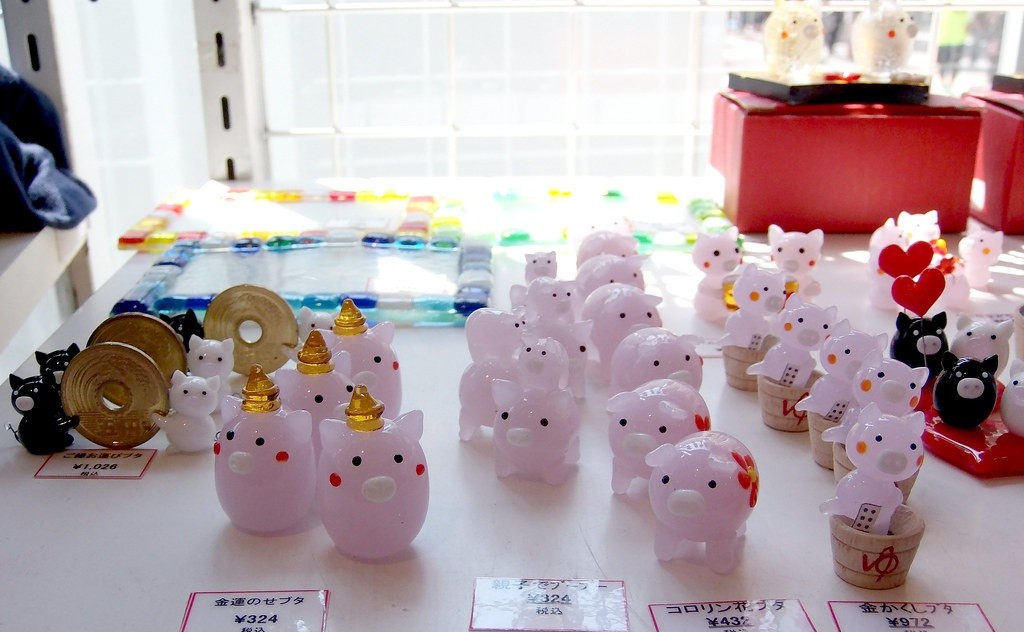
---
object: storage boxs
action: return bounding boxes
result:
[709,89,986,236]
[958,89,1024,240]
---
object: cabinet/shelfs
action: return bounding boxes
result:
[0,0,1024,631]
[0,1,100,377]
